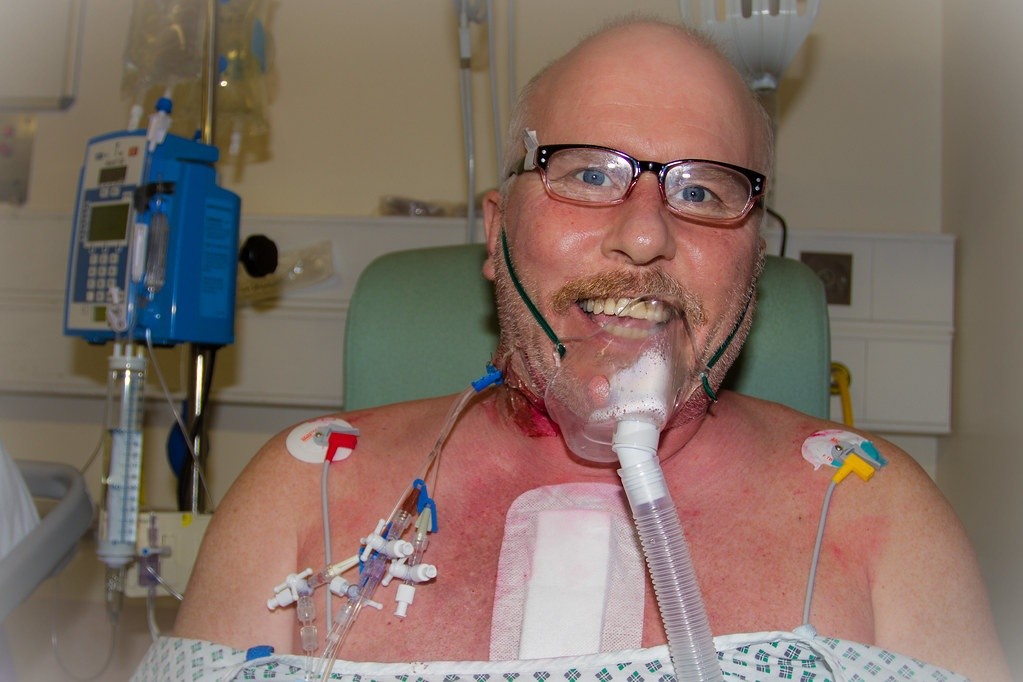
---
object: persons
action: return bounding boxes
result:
[133,9,1009,682]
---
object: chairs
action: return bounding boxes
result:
[342,242,834,421]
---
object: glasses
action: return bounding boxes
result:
[498,145,769,226]
